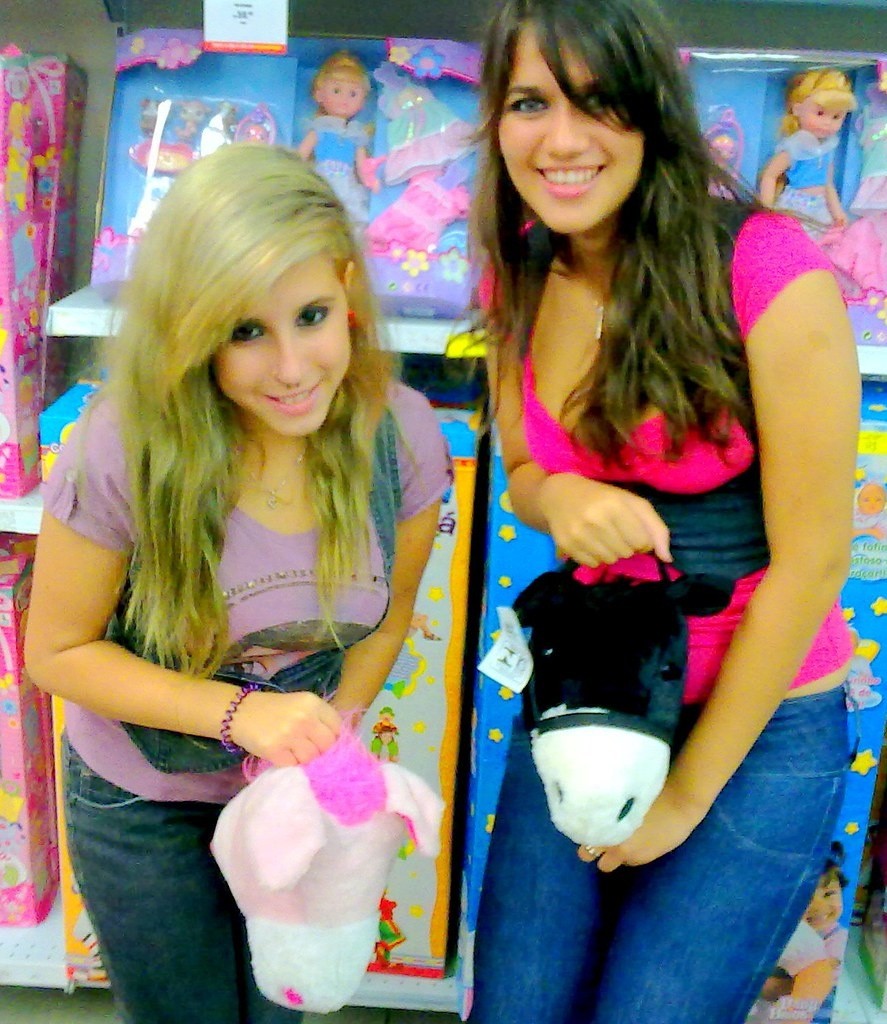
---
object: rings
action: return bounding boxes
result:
[584,843,601,858]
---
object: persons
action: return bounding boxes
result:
[745,854,844,1024]
[468,2,863,1024]
[760,68,857,235]
[296,55,373,226]
[24,143,456,1024]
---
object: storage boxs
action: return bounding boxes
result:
[0,25,887,1024]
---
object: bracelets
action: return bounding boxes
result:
[217,679,262,755]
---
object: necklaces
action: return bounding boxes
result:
[581,279,627,344]
[231,434,312,512]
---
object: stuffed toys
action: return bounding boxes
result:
[505,551,735,847]
[210,717,449,1013]
